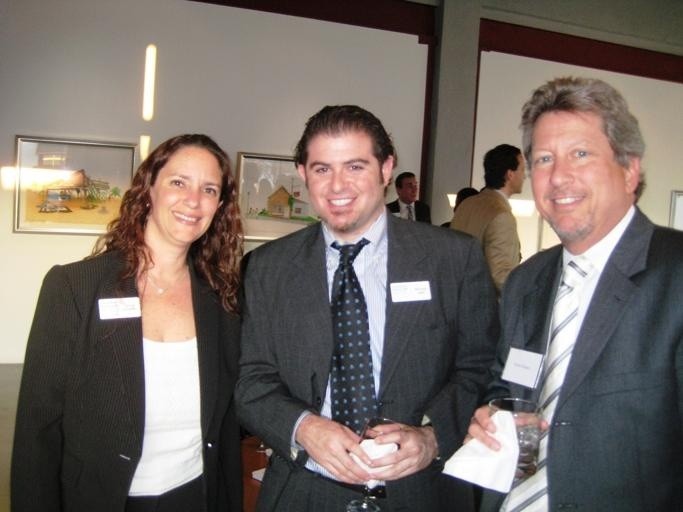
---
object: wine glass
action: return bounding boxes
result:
[346,415,402,512]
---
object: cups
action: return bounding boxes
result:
[488,396,542,487]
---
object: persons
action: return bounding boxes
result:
[10,134,244,510]
[449,144,526,305]
[386,172,432,225]
[440,188,479,228]
[232,105,500,512]
[461,75,683,511]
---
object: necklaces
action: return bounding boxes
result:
[140,265,190,295]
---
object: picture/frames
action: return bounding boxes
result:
[235,151,321,242]
[667,191,683,230]
[12,134,138,236]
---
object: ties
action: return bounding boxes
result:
[328,238,380,433]
[499,258,591,512]
[406,204,414,222]
